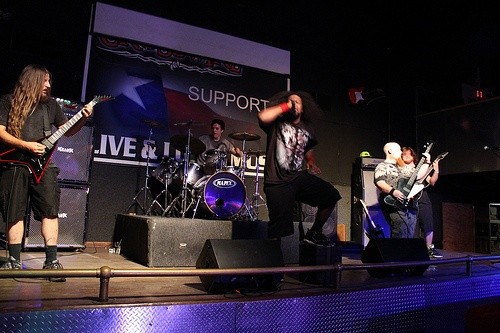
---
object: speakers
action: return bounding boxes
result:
[351,166,394,240]
[197,238,284,294]
[50,124,95,184]
[360,238,431,279]
[21,183,91,250]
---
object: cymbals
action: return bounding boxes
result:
[170,135,207,154]
[247,150,264,156]
[230,132,260,141]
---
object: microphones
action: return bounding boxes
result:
[290,99,297,113]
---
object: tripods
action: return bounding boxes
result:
[126,125,268,220]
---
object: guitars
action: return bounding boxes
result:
[383,142,433,213]
[0,94,116,183]
[359,199,385,238]
[408,152,448,199]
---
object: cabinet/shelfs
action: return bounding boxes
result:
[489,203,500,254]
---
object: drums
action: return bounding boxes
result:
[195,169,247,219]
[202,148,228,174]
[180,162,200,184]
[152,155,180,187]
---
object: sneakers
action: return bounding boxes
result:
[428,244,444,258]
[43,260,67,283]
[0,255,23,270]
[304,229,335,248]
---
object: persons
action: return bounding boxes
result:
[374,141,418,238]
[195,120,241,171]
[0,64,96,281]
[401,146,442,258]
[257,89,342,248]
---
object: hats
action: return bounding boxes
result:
[360,152,371,158]
